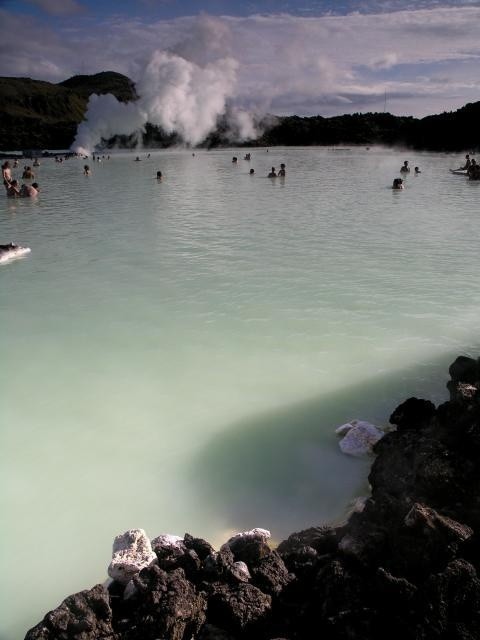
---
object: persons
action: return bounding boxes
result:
[156,171,164,179]
[269,164,286,178]
[249,168,255,174]
[135,153,150,161]
[400,161,421,173]
[1,152,111,198]
[460,155,480,180]
[232,153,251,162]
[392,178,404,189]
